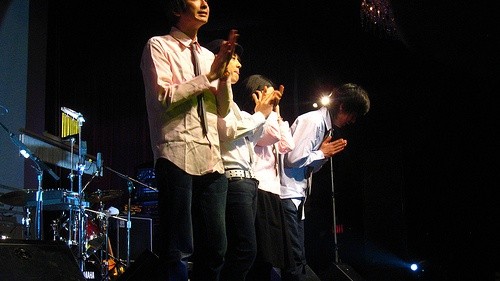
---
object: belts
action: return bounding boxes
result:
[226,169,253,178]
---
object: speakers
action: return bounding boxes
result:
[0,238,88,281]
[324,262,364,281]
[120,249,170,281]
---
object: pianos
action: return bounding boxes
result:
[0,188,90,273]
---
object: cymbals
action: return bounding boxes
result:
[86,190,127,203]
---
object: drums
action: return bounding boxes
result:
[59,213,100,246]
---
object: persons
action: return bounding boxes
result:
[207,39,274,281]
[86,177,120,218]
[141,0,238,281]
[241,75,295,281]
[280,82,371,281]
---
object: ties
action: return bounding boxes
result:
[307,124,331,190]
[190,43,208,135]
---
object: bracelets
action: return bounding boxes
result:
[221,72,230,79]
